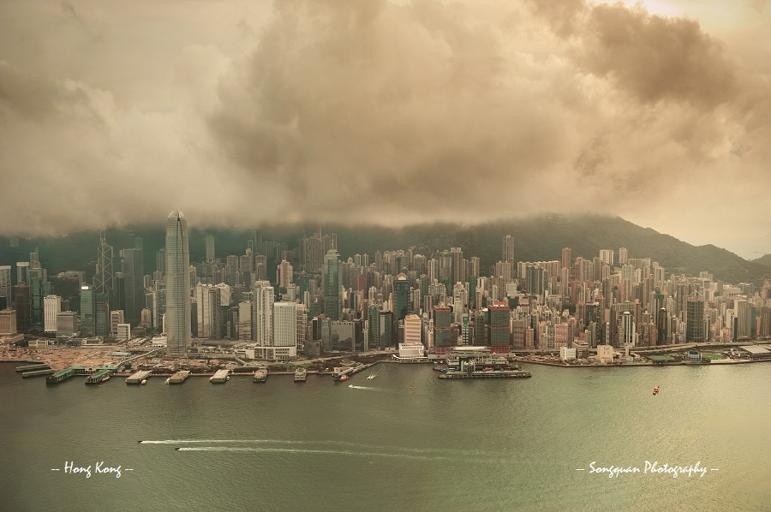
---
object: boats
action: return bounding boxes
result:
[47,367,308,385]
[432,346,532,379]
